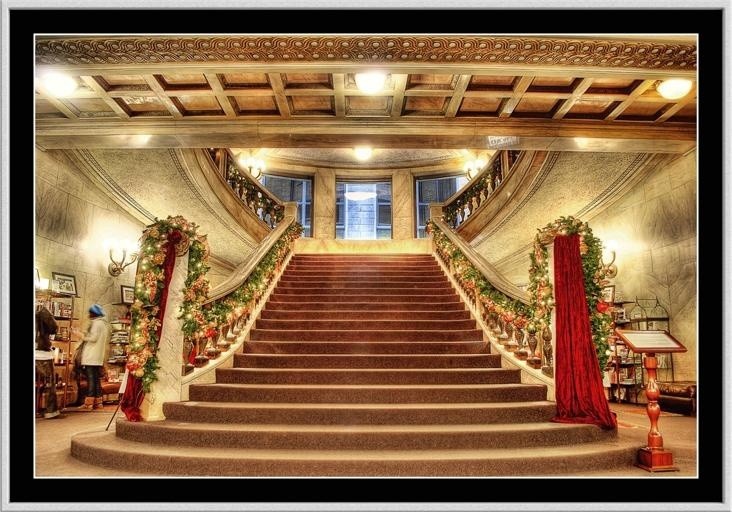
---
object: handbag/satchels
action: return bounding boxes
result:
[74,342,83,369]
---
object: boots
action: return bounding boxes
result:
[77,397,95,410]
[93,396,103,409]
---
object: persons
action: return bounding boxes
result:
[35,304,61,419]
[72,304,107,411]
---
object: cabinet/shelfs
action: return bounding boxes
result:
[602,299,643,403]
[107,301,135,382]
[36,289,81,418]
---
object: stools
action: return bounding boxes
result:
[654,380,697,416]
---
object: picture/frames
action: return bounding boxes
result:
[52,272,78,297]
[120,284,135,303]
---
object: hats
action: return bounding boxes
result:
[89,304,105,316]
